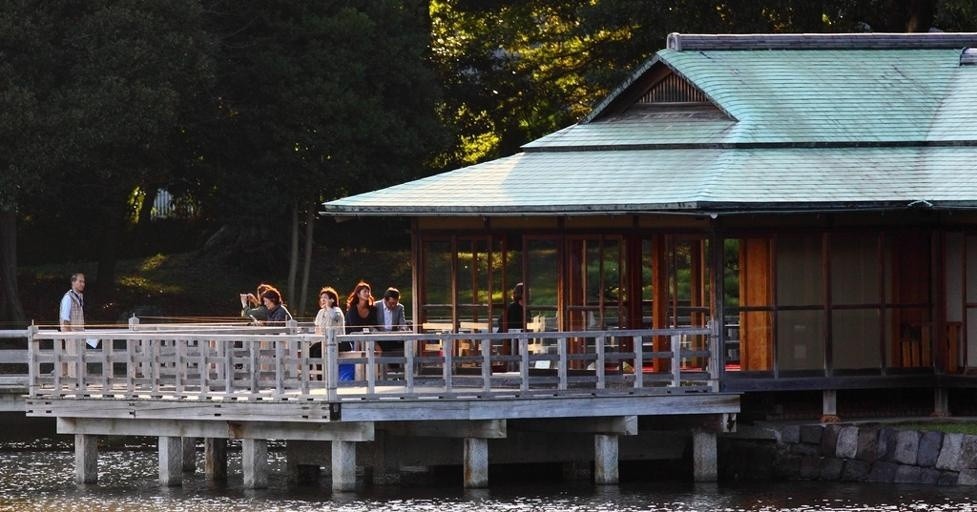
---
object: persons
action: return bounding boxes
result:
[239,282,407,382]
[58,272,91,399]
[497,281,533,356]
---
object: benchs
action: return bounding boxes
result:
[296,348,366,381]
[374,350,443,380]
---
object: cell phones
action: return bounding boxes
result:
[321,298,334,309]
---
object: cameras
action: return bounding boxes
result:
[247,295,249,299]
